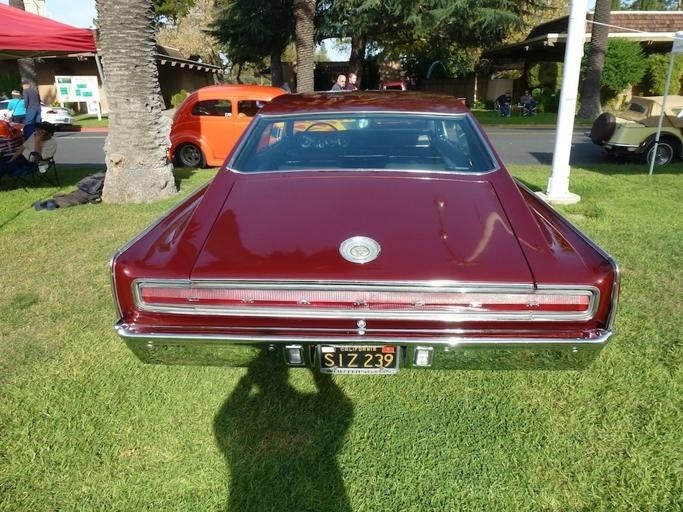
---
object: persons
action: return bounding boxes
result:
[521,90,537,117]
[343,72,359,90]
[496,90,512,118]
[5,90,27,123]
[1,121,56,178]
[21,80,41,125]
[281,80,291,95]
[332,74,346,91]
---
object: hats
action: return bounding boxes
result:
[32,121,56,134]
[11,78,32,96]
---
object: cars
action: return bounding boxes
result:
[106,89,623,377]
[169,85,294,168]
[586,94,683,165]
[382,81,407,92]
[1,98,73,139]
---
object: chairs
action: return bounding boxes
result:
[495,99,512,116]
[522,102,537,116]
[10,152,60,186]
